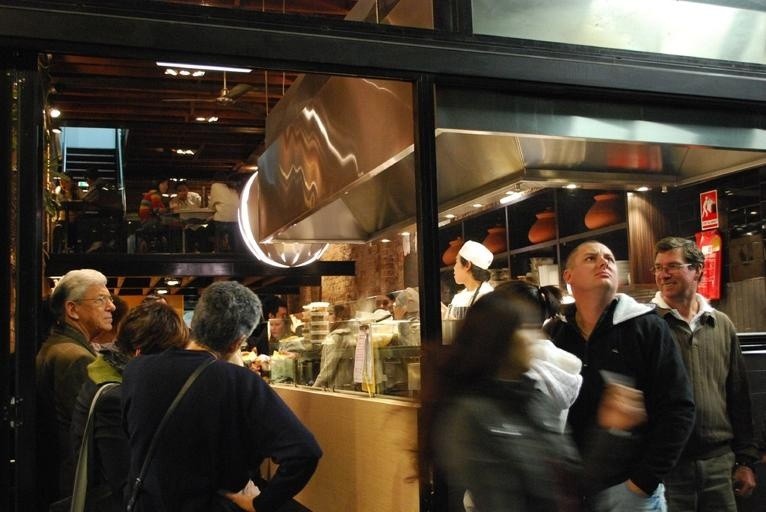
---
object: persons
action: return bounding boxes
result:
[312,291,421,402]
[34,269,324,512]
[432,236,759,512]
[35,166,240,256]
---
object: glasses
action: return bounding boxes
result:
[648,263,693,276]
[78,295,114,306]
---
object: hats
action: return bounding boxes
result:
[458,240,495,269]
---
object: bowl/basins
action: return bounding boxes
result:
[300,302,330,344]
[517,256,554,285]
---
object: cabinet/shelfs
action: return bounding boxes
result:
[438,181,676,303]
